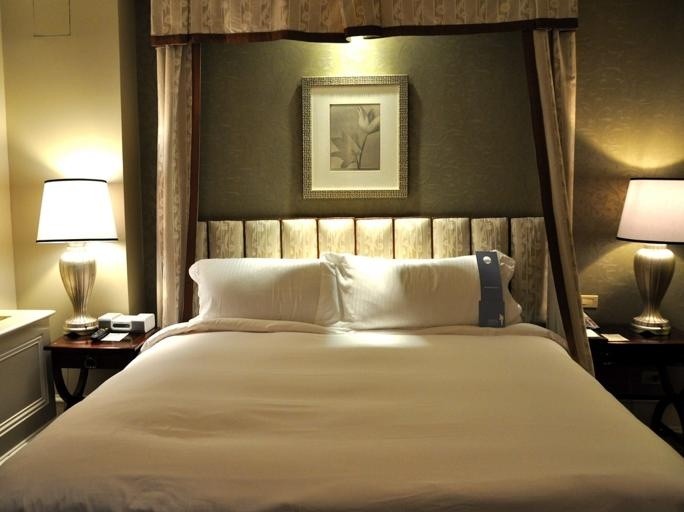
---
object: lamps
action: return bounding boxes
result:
[616,177,683,336]
[35,178,119,338]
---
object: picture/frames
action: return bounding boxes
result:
[300,74,410,202]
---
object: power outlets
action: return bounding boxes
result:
[640,372,663,386]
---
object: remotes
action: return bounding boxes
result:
[90,327,110,342]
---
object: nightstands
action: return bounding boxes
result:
[43,326,160,411]
[601,322,683,451]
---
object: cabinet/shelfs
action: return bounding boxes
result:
[1,309,55,461]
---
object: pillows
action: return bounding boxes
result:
[336,250,523,330]
[188,257,341,326]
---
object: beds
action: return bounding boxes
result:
[1,215,683,511]
